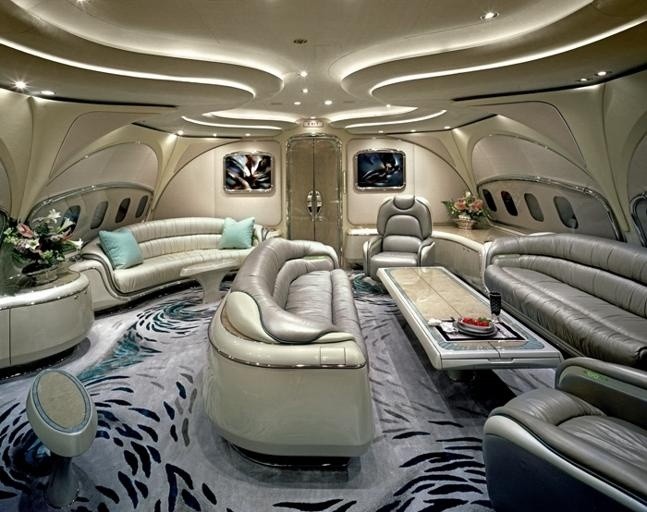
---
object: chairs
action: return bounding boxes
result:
[478,351,645,511]
[361,194,435,288]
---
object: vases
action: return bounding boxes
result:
[19,264,60,285]
[452,215,477,230]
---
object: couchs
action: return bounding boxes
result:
[200,234,375,473]
[74,212,278,313]
[478,230,646,373]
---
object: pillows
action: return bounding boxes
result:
[217,215,254,250]
[97,224,144,272]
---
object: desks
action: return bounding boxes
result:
[179,258,238,304]
[24,364,100,507]
[375,261,565,404]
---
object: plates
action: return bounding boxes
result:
[457,320,497,337]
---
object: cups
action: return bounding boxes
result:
[489,291,500,315]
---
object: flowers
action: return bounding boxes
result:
[446,187,493,220]
[2,209,91,264]
